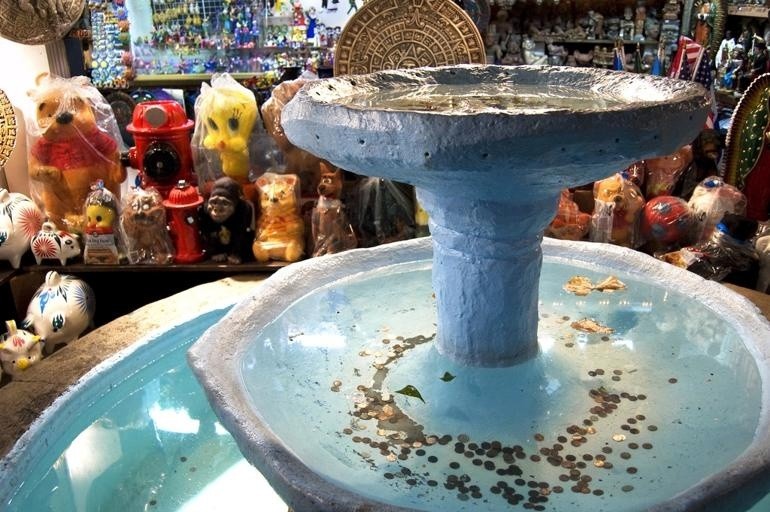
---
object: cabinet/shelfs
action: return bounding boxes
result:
[40,0,769,259]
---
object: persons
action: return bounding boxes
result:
[709,36,767,98]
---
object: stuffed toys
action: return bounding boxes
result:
[0,36,770,381]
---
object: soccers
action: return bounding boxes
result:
[644,194,691,247]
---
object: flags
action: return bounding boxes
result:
[614,35,718,130]
